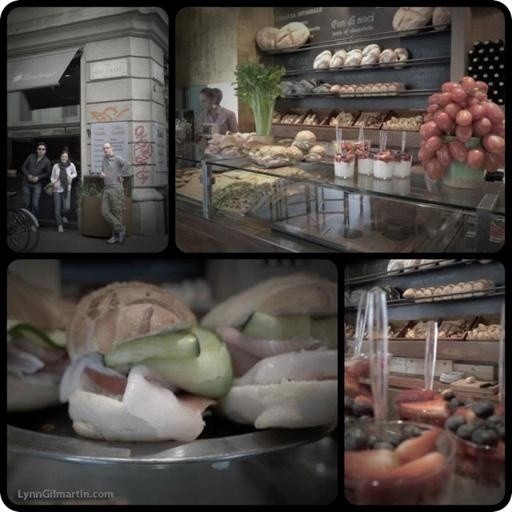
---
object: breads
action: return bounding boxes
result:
[431,7,451,31]
[255,26,280,51]
[272,110,319,126]
[275,22,309,49]
[330,81,405,99]
[312,43,409,73]
[286,130,327,162]
[402,278,495,304]
[387,257,490,276]
[392,7,434,37]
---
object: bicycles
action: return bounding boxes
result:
[8,191,39,253]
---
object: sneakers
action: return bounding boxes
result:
[30,225,37,232]
[57,225,63,232]
[63,217,69,224]
[107,236,117,244]
[119,225,127,244]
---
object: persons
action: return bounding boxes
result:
[99,141,130,246]
[50,150,78,232]
[194,87,240,141]
[22,142,52,219]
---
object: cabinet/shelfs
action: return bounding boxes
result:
[261,25,451,147]
[343,257,507,405]
[176,138,506,254]
[82,173,132,237]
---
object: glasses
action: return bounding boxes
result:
[37,148,46,151]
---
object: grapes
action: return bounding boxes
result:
[417,76,505,180]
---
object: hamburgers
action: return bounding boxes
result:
[199,272,338,429]
[7,269,77,412]
[68,280,199,440]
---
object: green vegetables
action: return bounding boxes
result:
[232,60,286,137]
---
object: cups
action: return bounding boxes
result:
[332,139,413,181]
[345,352,505,506]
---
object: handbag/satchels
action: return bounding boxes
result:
[43,183,54,196]
